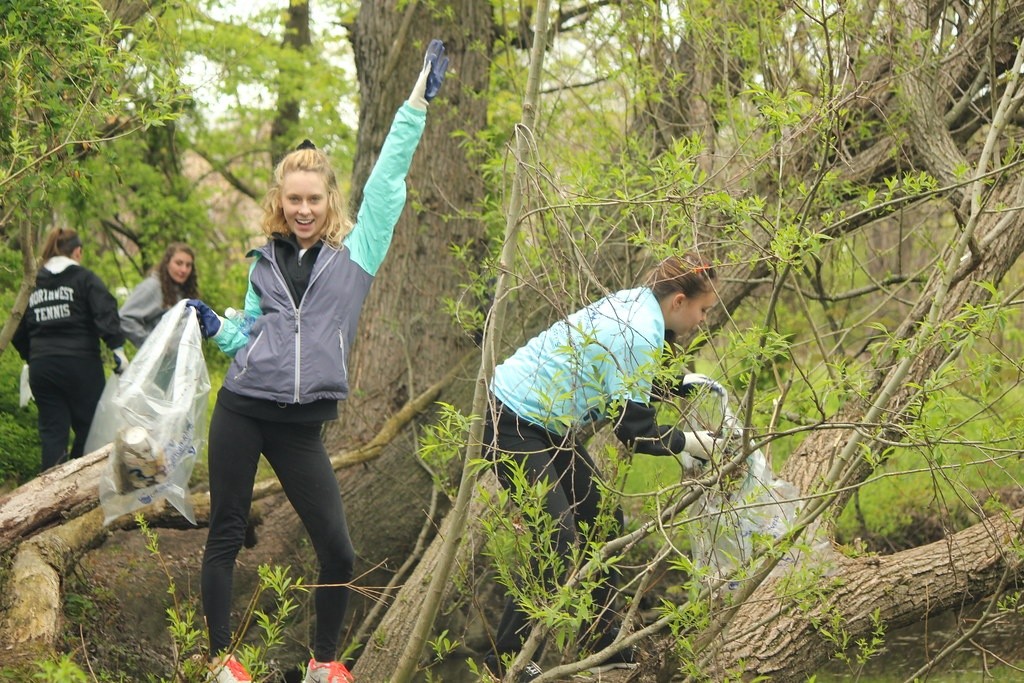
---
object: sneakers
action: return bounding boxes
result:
[303,658,353,683]
[207,659,252,683]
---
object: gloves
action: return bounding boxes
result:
[186,299,224,338]
[113,345,129,374]
[683,430,723,465]
[408,39,449,109]
[682,373,724,397]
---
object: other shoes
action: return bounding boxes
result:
[586,638,643,675]
[485,659,543,683]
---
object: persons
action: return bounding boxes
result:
[481,252,725,683]
[10,227,129,473]
[185,38,450,683]
[118,242,200,420]
